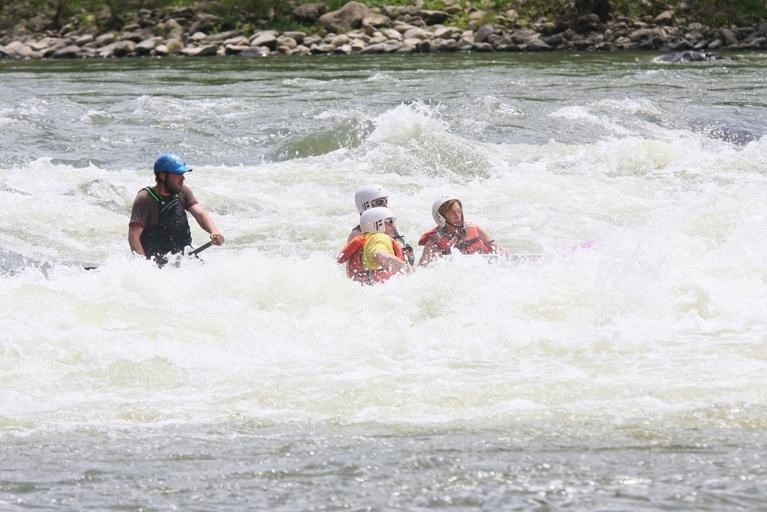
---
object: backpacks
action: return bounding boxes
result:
[138,187,192,254]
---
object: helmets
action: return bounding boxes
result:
[154,154,192,175]
[355,185,396,234]
[432,197,464,229]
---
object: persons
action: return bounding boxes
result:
[128,153,225,271]
[336,184,517,287]
